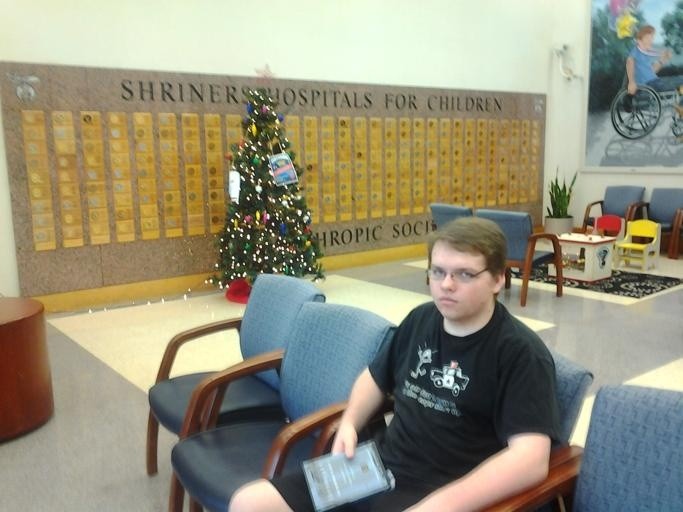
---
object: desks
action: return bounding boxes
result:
[0,296,56,444]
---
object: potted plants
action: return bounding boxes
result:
[544,165,577,235]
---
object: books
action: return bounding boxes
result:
[302,438,391,510]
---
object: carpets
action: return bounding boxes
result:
[510,257,681,300]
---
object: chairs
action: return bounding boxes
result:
[473,208,563,306]
[426,202,472,282]
[616,218,661,270]
[144,272,324,512]
[316,345,592,512]
[483,383,682,512]
[168,301,395,512]
[630,187,681,258]
[581,184,645,236]
[593,214,625,265]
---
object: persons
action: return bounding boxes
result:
[225,215,561,509]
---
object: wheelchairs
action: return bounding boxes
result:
[610,63,683,141]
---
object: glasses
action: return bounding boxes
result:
[426,266,487,282]
